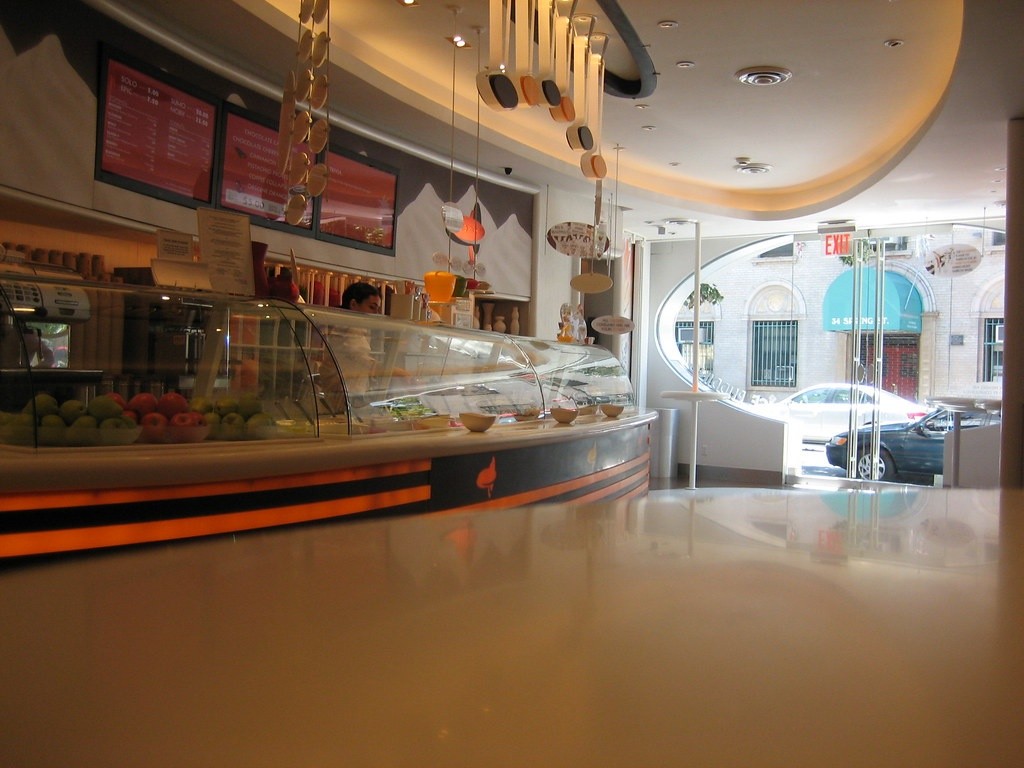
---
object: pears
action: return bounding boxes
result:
[0,393,139,446]
[188,393,272,440]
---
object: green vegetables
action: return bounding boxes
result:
[384,398,434,420]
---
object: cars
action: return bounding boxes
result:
[751,382,935,443]
[825,402,1000,482]
[368,371,615,412]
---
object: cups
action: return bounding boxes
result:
[93,254,104,275]
[79,253,92,273]
[35,248,49,263]
[51,249,63,265]
[99,272,112,282]
[63,251,76,271]
[3,241,16,256]
[17,244,31,258]
[113,276,124,284]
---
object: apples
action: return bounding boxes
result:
[103,391,210,444]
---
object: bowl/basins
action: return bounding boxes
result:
[459,412,496,432]
[550,407,578,424]
[514,414,537,422]
[600,405,624,417]
[576,405,597,416]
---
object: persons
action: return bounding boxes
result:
[319,283,419,415]
